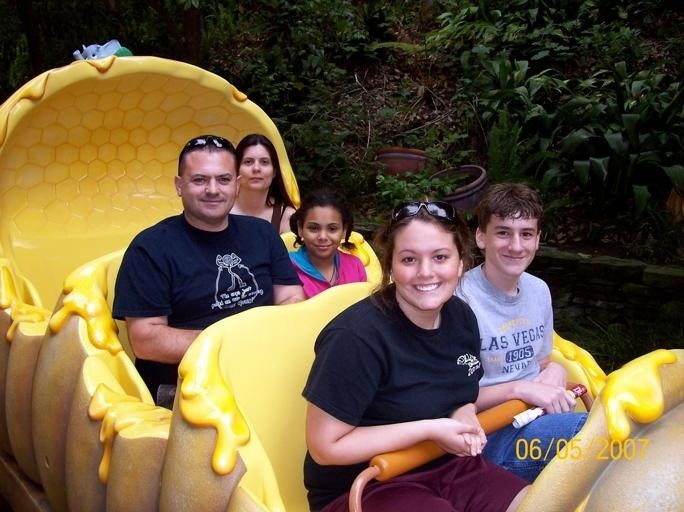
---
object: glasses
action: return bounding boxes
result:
[183,135,230,152]
[390,200,458,223]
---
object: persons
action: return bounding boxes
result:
[109,133,303,416]
[456,181,587,482]
[277,193,367,303]
[227,134,294,238]
[299,194,535,511]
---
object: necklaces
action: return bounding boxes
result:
[329,264,336,283]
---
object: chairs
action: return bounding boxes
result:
[0,53,684,512]
